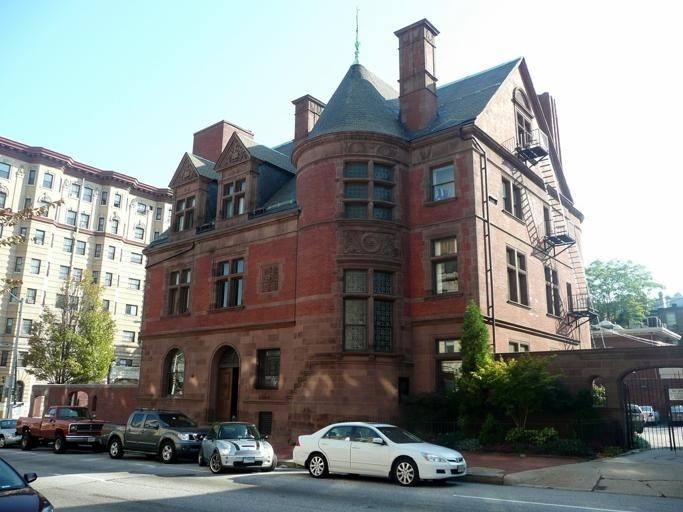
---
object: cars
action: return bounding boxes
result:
[292,421,467,486]
[669,405,683,426]
[0,457,56,512]
[639,406,663,426]
[198,421,277,474]
[0,418,23,448]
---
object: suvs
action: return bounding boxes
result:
[627,404,646,433]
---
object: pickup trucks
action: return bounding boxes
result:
[16,405,112,454]
[101,405,211,463]
